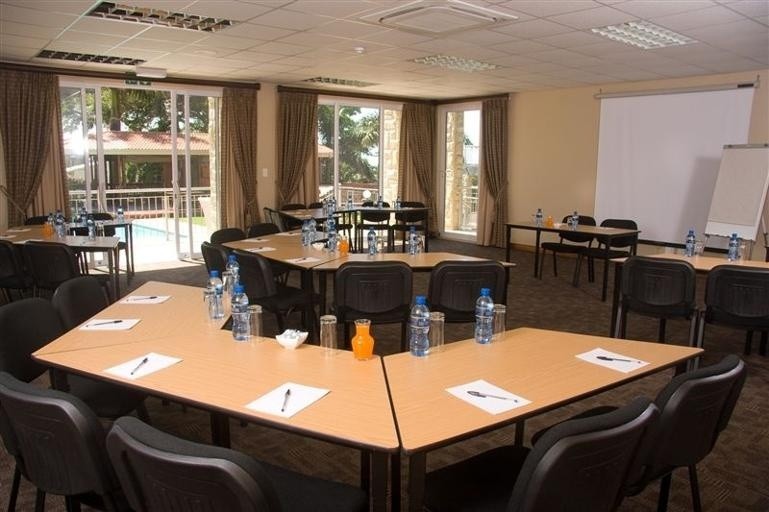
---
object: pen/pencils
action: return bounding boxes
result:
[127,296,158,301]
[255,238,262,240]
[86,320,123,327]
[250,247,262,251]
[131,356,148,376]
[467,390,518,403]
[3,235,9,236]
[282,389,290,411]
[296,257,306,262]
[597,357,634,362]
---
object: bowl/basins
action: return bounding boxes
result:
[274,329,308,350]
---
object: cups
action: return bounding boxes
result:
[488,303,506,342]
[319,313,339,358]
[244,304,264,344]
[694,240,704,256]
[738,241,747,261]
[426,311,446,355]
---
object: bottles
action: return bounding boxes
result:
[116,205,124,223]
[472,287,495,344]
[207,269,223,320]
[351,318,375,361]
[534,207,580,232]
[320,190,400,217]
[39,204,104,242]
[409,295,430,357]
[224,254,240,301]
[727,234,739,263]
[230,283,250,342]
[300,213,423,257]
[684,229,694,256]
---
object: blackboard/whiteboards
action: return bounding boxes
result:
[703,143,769,241]
